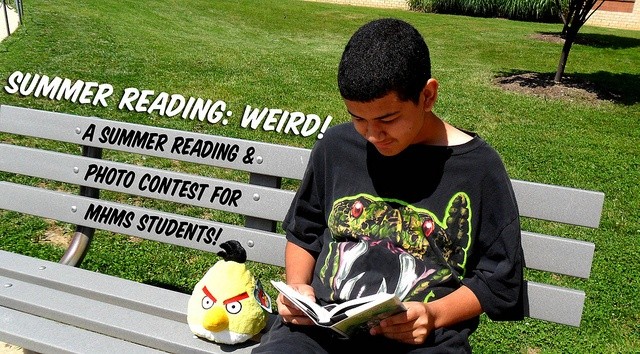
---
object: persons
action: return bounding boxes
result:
[251,18,525,353]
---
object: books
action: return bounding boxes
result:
[269,278,408,339]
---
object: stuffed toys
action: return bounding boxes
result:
[186,238,273,345]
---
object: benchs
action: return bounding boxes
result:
[0,102,605,353]
